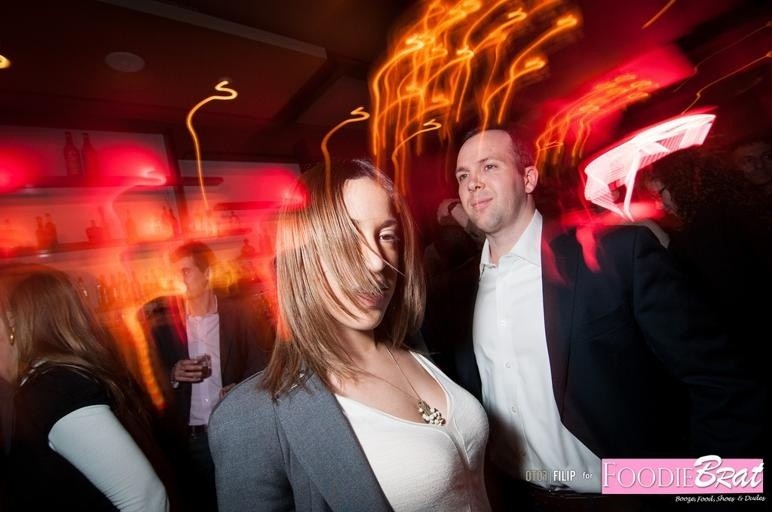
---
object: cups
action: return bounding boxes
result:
[190,351,210,379]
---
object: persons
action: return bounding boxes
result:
[2,260,190,510]
[148,240,271,511]
[724,128,772,199]
[396,116,743,511]
[622,144,770,260]
[202,155,499,512]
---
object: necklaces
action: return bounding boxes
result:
[334,342,447,427]
